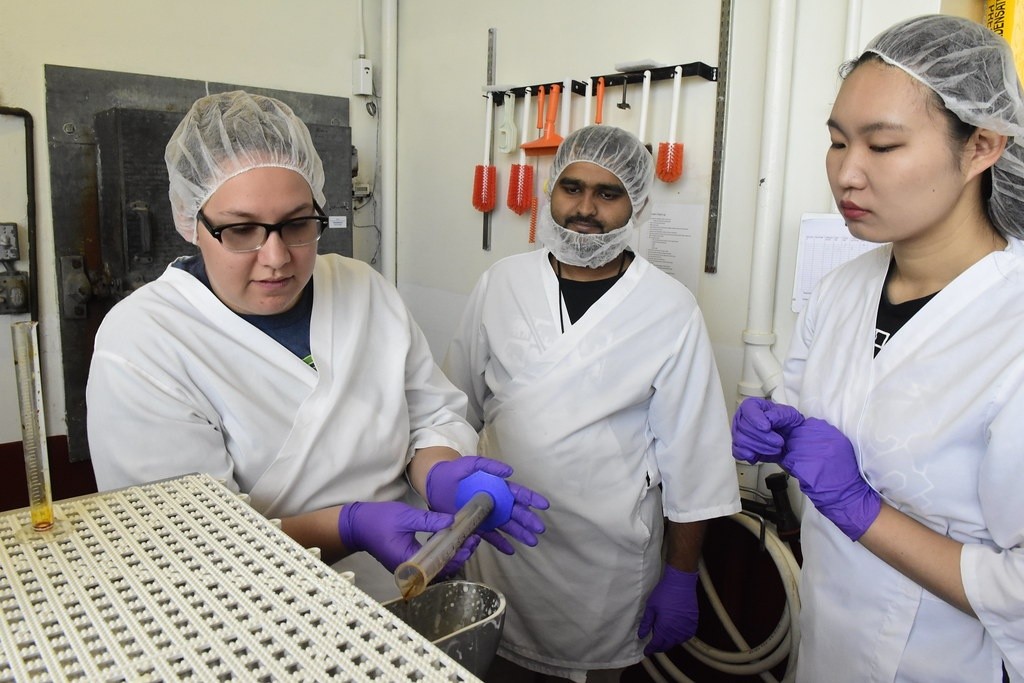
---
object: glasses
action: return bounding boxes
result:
[197,197,329,253]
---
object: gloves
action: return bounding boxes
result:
[425,455,550,556]
[731,396,806,466]
[780,417,881,542]
[339,501,480,586]
[638,561,698,656]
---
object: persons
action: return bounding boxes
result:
[87,90,551,589]
[730,14,1024,683]
[443,127,742,681]
